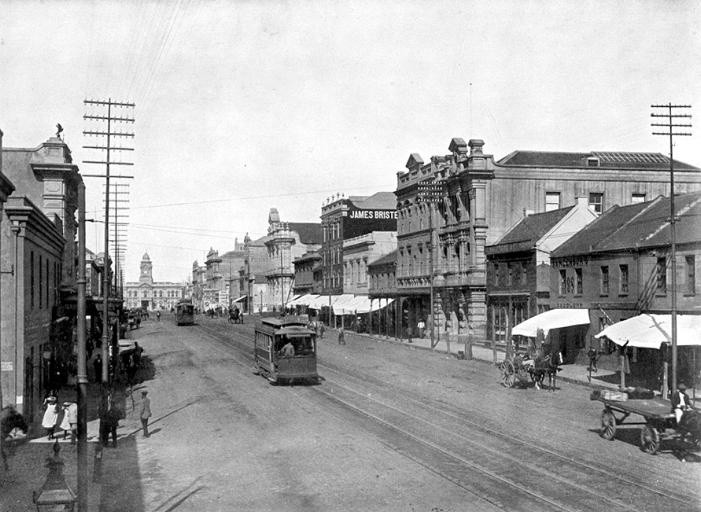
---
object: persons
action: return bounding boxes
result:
[671,383,695,425]
[105,390,153,446]
[312,320,345,345]
[42,396,79,444]
[589,345,598,371]
[417,318,426,338]
[193,306,243,322]
[42,305,161,386]
[279,341,295,356]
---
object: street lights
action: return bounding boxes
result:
[32,435,79,512]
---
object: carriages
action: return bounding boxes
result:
[228,308,243,324]
[496,335,564,392]
[590,396,701,463]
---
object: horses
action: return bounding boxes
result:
[534,348,565,393]
[677,408,701,465]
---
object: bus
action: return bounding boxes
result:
[252,315,320,387]
[174,299,194,326]
